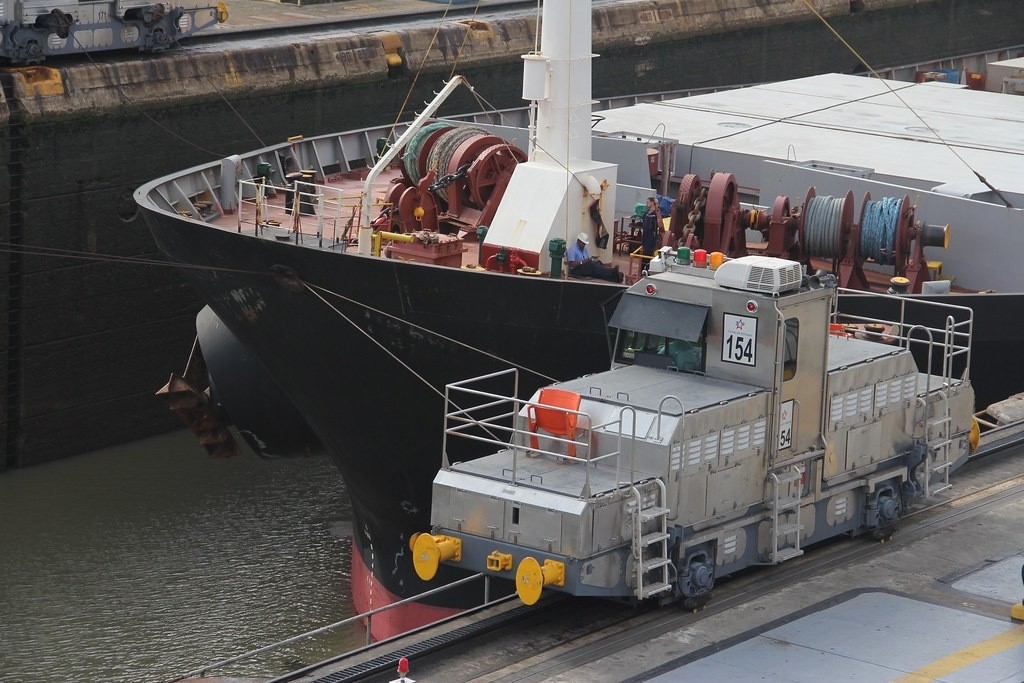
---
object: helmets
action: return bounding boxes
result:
[577,233,589,244]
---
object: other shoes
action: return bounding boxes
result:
[613,265,624,283]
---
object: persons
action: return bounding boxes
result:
[640,196,661,277]
[566,232,625,283]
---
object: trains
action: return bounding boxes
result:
[407,243,980,617]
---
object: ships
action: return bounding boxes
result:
[130,43,1024,648]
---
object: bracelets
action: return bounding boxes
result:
[580,261,582,265]
